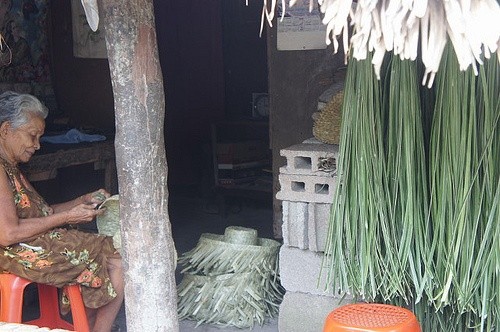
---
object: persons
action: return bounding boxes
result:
[0,91,123,332]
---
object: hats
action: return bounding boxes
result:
[96,195,177,272]
[177,226,285,332]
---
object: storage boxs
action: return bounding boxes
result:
[217,160,264,181]
[215,138,268,165]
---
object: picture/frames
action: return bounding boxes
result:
[71,0,108,59]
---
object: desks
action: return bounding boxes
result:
[18,123,118,197]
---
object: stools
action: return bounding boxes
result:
[0,272,89,332]
[323,304,421,332]
